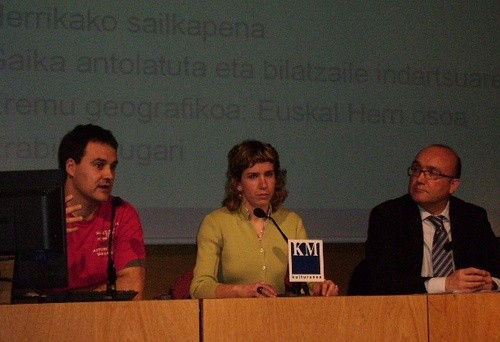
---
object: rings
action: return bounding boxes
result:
[257,286,263,294]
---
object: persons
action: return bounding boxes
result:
[348,144,500,296]
[189,140,338,297]
[41,123,148,302]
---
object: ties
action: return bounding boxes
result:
[427,215,454,277]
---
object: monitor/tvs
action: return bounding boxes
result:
[0,169,66,302]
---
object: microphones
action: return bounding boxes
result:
[100,194,128,300]
[253,207,312,296]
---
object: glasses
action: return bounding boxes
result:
[407,166,457,180]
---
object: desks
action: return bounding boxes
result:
[202,292,500,342]
[0,300,200,342]
